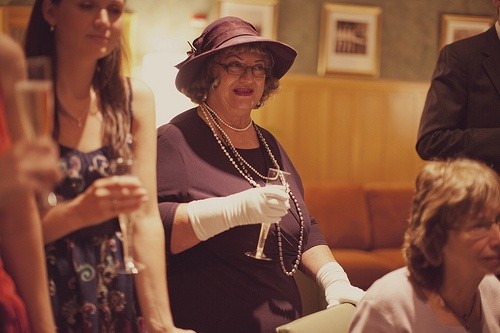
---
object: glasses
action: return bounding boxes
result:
[445,216,500,240]
[209,60,266,77]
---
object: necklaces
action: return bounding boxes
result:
[201,101,303,276]
[57,95,99,126]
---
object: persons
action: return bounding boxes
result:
[415,0,500,173]
[22,0,196,333]
[348,157,500,333]
[0,33,59,333]
[157,17,365,333]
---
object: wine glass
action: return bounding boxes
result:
[9,54,64,209]
[109,158,145,275]
[244,167,292,261]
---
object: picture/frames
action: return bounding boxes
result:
[317,2,383,77]
[224,0,281,39]
[438,12,496,47]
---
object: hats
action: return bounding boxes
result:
[174,16,298,104]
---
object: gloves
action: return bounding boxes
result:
[187,183,290,241]
[316,262,365,308]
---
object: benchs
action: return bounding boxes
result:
[292,183,417,311]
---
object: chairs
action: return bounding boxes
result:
[275,301,359,333]
